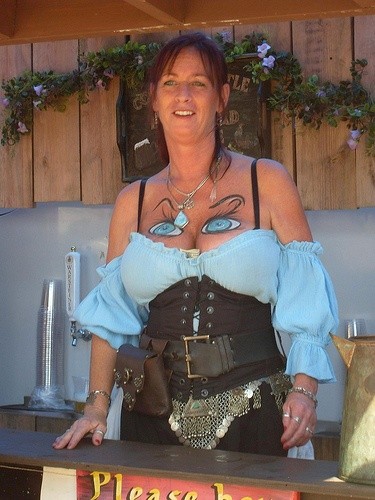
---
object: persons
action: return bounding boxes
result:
[53,30,341,461]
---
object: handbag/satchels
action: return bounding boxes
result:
[328,332,375,486]
[113,335,169,418]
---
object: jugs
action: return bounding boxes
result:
[327,330,375,486]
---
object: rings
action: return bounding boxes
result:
[283,413,290,417]
[292,416,301,424]
[66,429,70,431]
[306,426,313,433]
[95,428,104,436]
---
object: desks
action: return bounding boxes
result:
[0,428,375,500]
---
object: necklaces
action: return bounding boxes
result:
[166,153,223,210]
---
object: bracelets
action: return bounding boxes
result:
[288,385,319,408]
[86,390,111,406]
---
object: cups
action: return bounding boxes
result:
[347,320,364,339]
[35,279,65,410]
[71,375,89,412]
